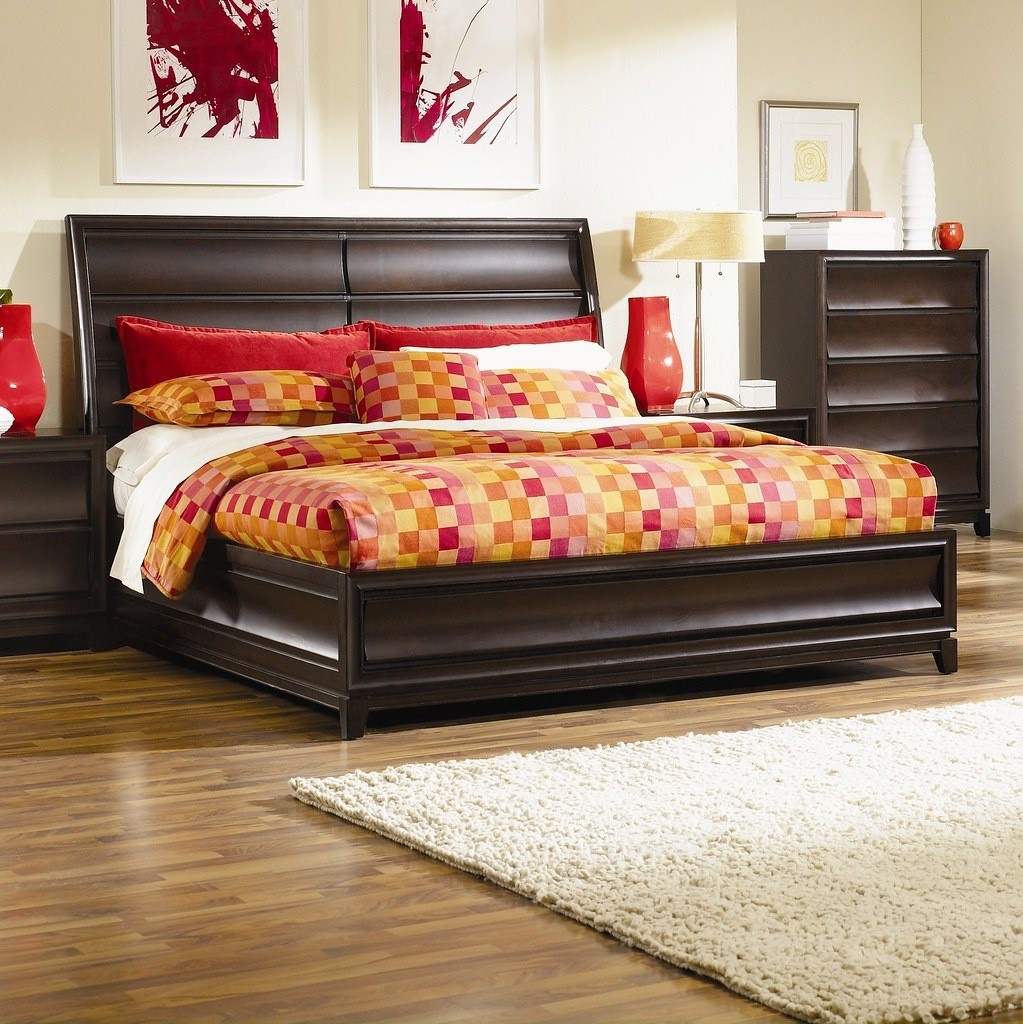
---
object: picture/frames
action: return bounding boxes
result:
[759,99,861,221]
[113,0,306,188]
[367,0,546,191]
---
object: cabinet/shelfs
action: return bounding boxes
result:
[760,244,992,537]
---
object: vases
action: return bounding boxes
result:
[621,297,684,413]
[901,123,937,250]
[1,302,46,434]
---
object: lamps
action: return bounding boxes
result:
[631,209,768,412]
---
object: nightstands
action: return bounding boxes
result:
[641,404,818,449]
[0,428,109,656]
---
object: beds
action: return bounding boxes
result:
[62,213,959,742]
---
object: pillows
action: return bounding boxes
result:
[106,304,642,437]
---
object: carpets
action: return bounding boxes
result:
[284,695,1023,1024]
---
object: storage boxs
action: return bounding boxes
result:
[739,379,776,408]
